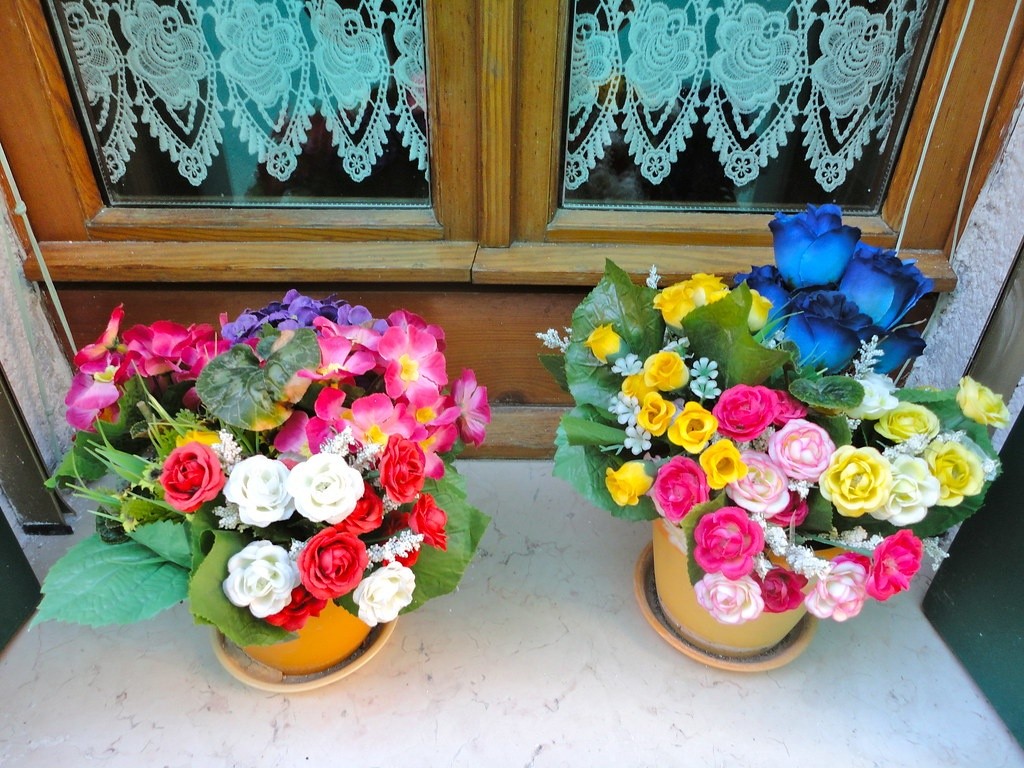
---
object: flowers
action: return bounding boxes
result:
[534,202,1013,623]
[28,290,493,647]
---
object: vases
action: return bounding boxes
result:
[208,597,399,691]
[635,518,818,672]
